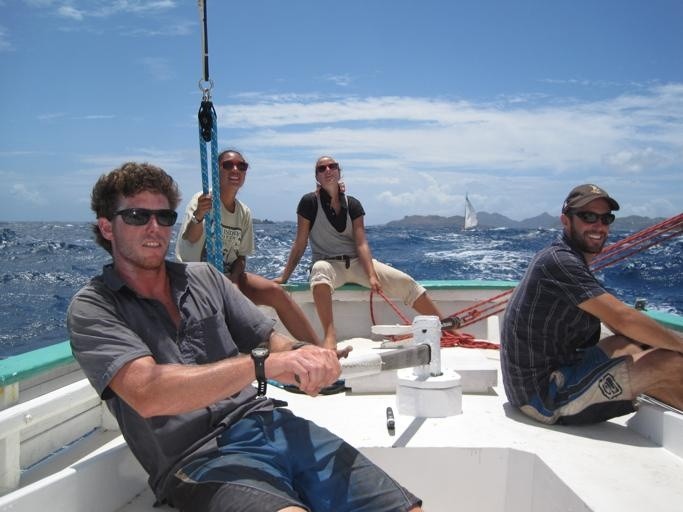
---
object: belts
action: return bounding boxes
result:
[331,255,350,269]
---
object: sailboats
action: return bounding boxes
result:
[461,193,479,232]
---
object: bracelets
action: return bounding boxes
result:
[292,340,312,349]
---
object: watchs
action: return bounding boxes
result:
[191,211,204,225]
[250,347,269,397]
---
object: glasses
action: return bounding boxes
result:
[317,163,339,172]
[568,212,616,225]
[113,208,177,226]
[222,161,248,171]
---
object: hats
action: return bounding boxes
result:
[562,184,619,213]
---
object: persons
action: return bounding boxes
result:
[174,150,353,360]
[67,162,424,512]
[272,156,443,350]
[499,184,683,425]
